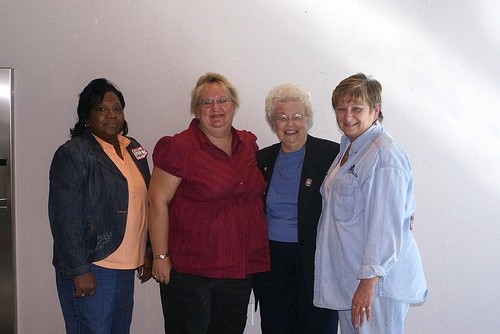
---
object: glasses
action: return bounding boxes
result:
[273,113,309,122]
[196,97,234,107]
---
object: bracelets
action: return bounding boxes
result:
[153,253,171,259]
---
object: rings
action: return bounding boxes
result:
[361,307,365,311]
[81,294,85,297]
[154,275,157,278]
[367,307,371,310]
[353,306,355,308]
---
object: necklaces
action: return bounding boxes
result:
[278,145,305,180]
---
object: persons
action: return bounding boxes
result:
[312,71,428,334]
[253,80,415,334]
[147,73,270,334]
[48,77,153,334]
